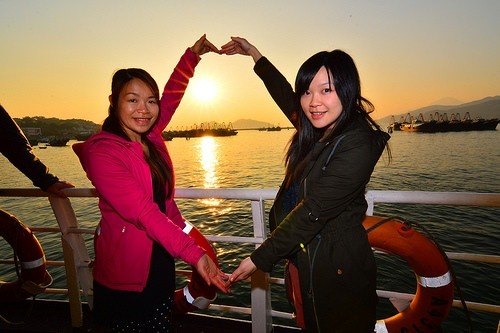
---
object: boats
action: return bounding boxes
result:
[48,137,69,147]
[168,121,239,138]
[75,131,93,141]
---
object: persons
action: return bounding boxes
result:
[72,34,228,333]
[219,37,391,333]
[0,104,75,198]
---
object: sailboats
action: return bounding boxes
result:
[258,123,282,131]
[387,112,500,133]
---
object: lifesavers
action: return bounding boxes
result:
[284,216,455,333]
[173,218,218,316]
[0,210,47,304]
